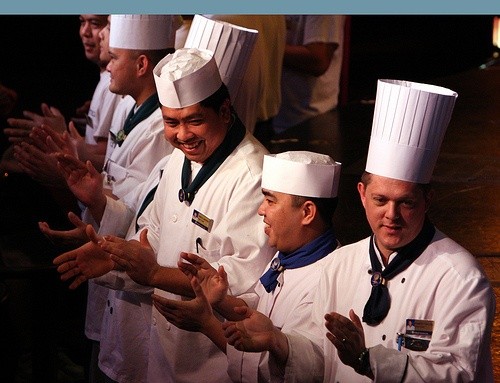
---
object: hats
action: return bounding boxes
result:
[108,14,173,49]
[185,14,258,97]
[261,151,342,197]
[365,78,458,184]
[152,48,223,109]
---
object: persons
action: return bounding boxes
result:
[223,79,496,383]
[193,211,198,221]
[406,319,415,330]
[0,14,345,383]
[151,151,342,383]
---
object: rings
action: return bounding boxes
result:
[342,339,346,342]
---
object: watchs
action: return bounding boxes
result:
[356,350,370,375]
